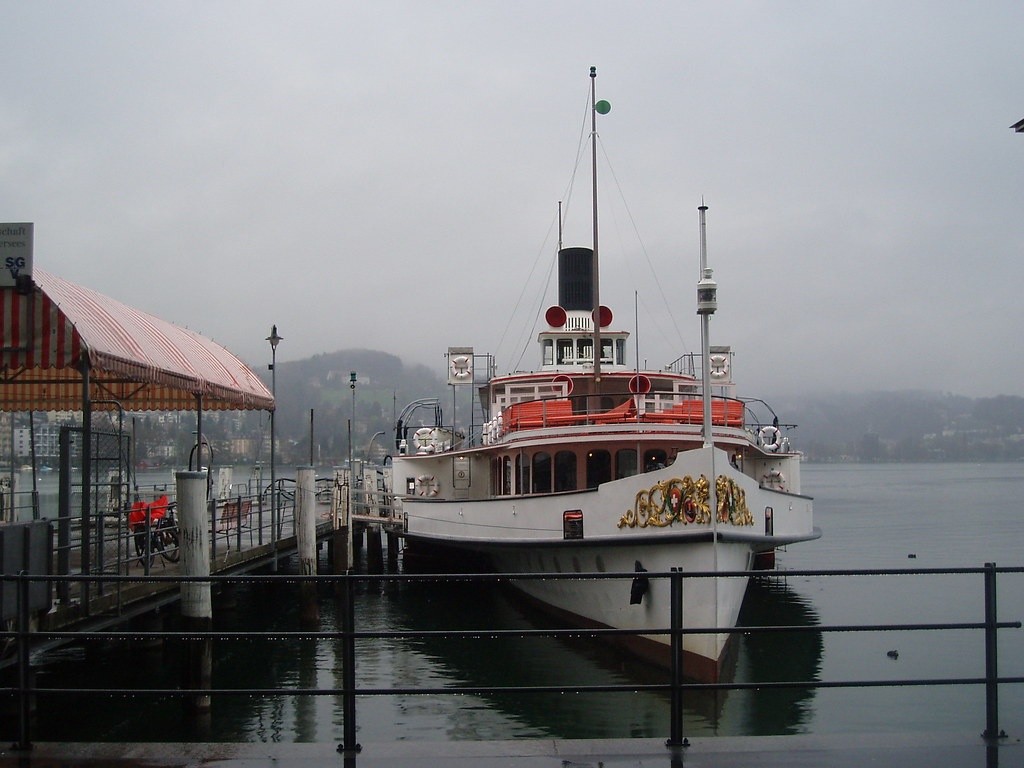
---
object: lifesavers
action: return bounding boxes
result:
[709,355,729,377]
[758,426,782,450]
[413,427,437,452]
[762,470,786,491]
[451,356,472,379]
[416,474,440,497]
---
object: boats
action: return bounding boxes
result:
[19,465,79,472]
[195,467,215,473]
[392,62,826,692]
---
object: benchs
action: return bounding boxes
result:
[502,397,743,430]
[208,500,253,548]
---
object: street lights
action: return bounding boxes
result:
[264,325,285,549]
[348,370,360,514]
[192,430,213,503]
[368,432,386,463]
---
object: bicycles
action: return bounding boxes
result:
[134,504,179,570]
